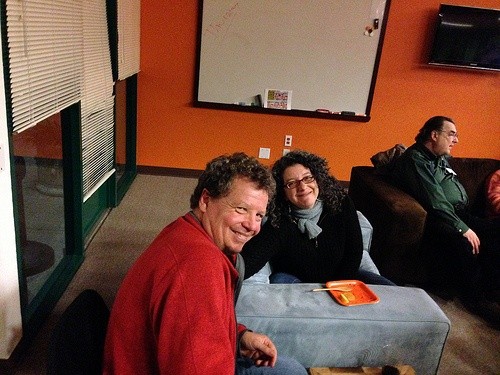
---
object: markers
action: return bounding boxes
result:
[315,109,366,117]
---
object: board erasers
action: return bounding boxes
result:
[255,94,262,108]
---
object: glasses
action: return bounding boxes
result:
[437,129,458,138]
[284,175,317,190]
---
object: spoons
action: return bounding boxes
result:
[312,287,353,292]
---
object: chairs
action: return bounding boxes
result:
[47,287,110,375]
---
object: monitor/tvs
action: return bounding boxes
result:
[427,3,500,72]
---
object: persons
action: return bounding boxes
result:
[485,169,500,213]
[393,116,500,330]
[103,153,308,375]
[268,150,397,287]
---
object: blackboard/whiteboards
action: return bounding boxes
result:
[194,1,392,123]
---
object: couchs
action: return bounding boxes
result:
[236,202,449,375]
[348,158,500,292]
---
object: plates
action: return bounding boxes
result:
[326,280,380,307]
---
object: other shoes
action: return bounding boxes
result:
[475,294,500,326]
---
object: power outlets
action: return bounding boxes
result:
[285,134,292,146]
